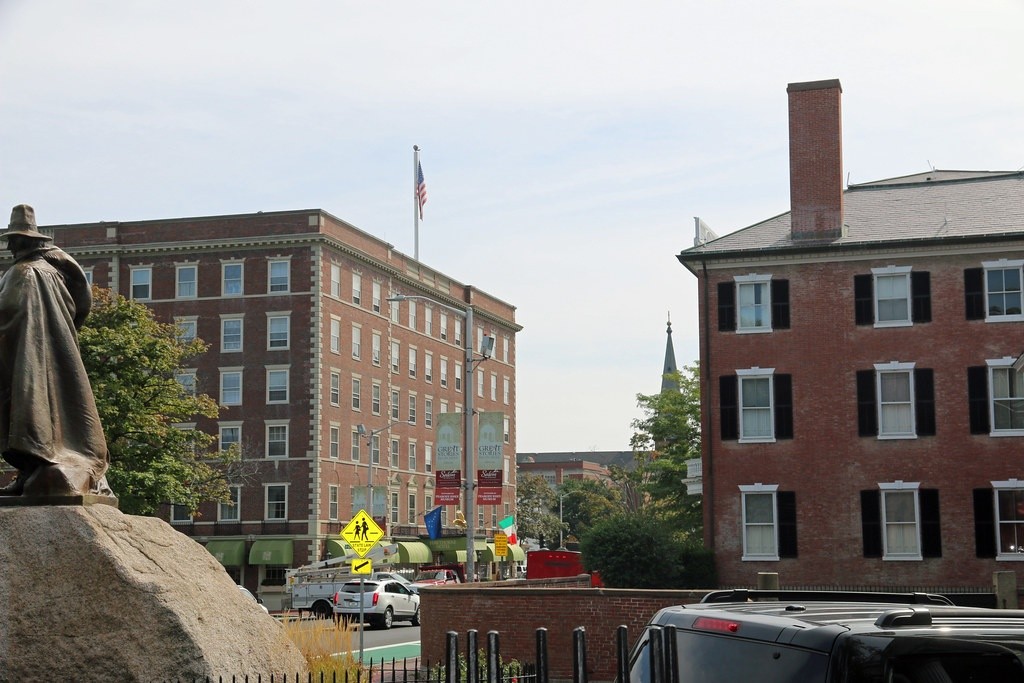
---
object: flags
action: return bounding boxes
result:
[416,159,428,221]
[423,507,444,541]
[497,515,519,545]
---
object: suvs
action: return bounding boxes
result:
[332,578,421,629]
[614,592,1024,683]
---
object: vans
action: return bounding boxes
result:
[517,565,527,578]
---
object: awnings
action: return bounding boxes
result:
[324,539,400,566]
[394,542,433,564]
[248,540,294,566]
[485,543,526,563]
[448,550,478,562]
[202,540,244,566]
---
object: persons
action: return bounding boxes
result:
[0,204,117,504]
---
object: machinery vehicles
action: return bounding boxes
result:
[285,543,412,619]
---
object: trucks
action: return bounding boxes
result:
[526,550,605,587]
[413,564,468,585]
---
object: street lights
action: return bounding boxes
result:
[560,490,580,549]
[385,294,475,581]
